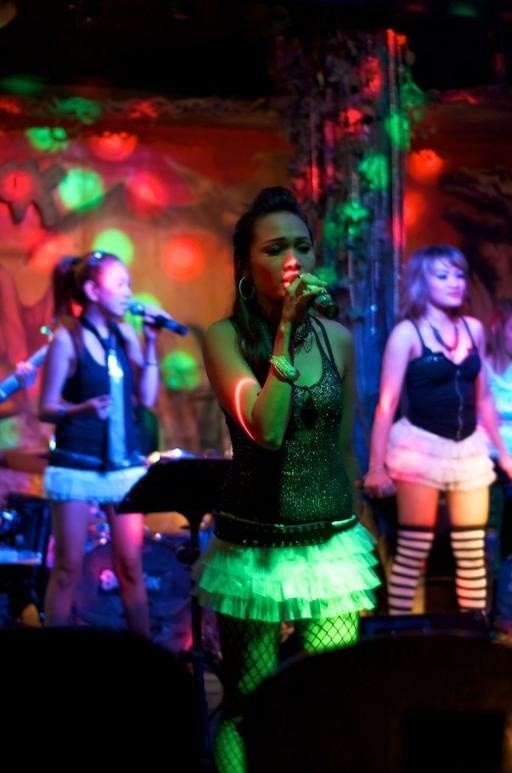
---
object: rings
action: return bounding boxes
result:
[297,275,302,278]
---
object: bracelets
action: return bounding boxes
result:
[266,353,302,382]
[56,402,71,421]
[141,358,159,366]
[367,464,387,474]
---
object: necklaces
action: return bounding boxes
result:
[284,318,314,355]
[423,316,459,352]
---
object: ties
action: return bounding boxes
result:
[78,312,127,468]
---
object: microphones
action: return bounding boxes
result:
[129,302,188,336]
[306,284,341,320]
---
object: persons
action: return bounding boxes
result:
[187,185,383,772]
[365,239,512,626]
[36,246,161,640]
